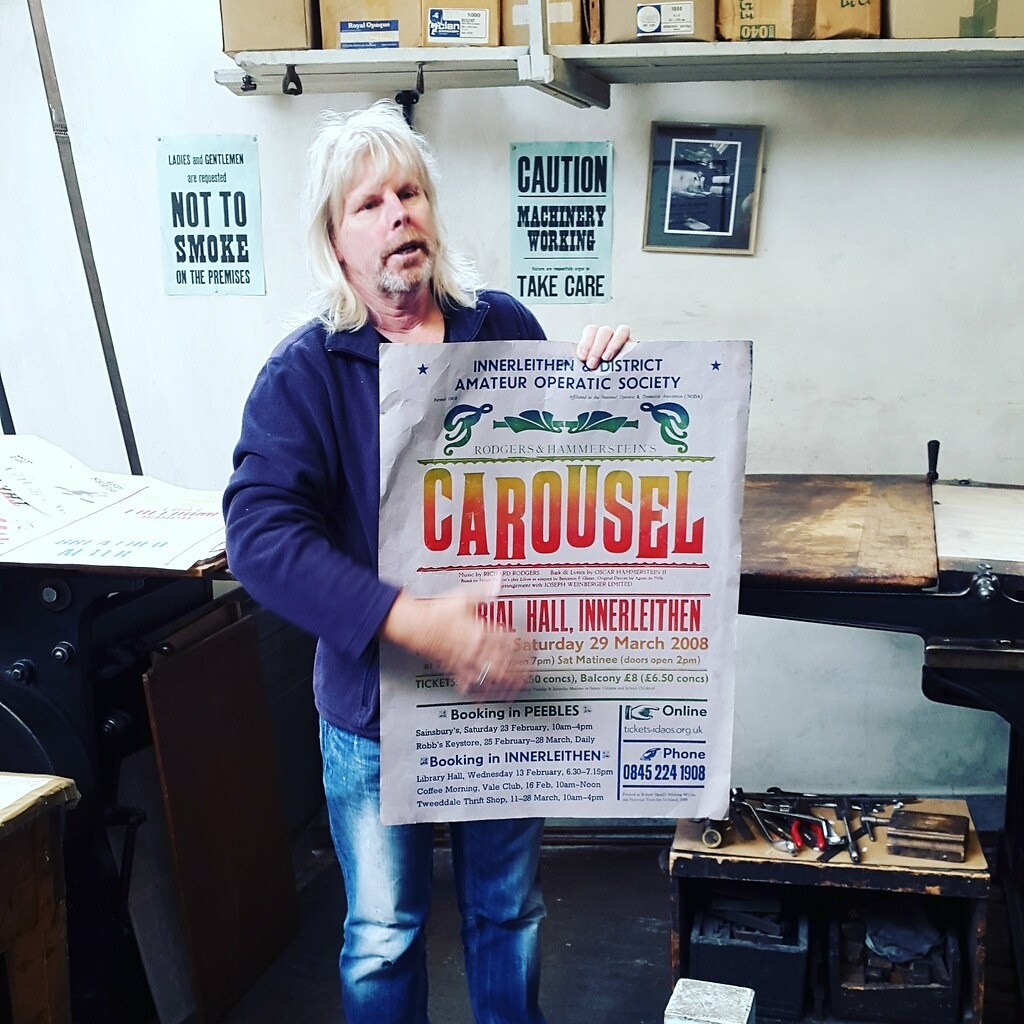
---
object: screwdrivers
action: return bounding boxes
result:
[836,796,860,863]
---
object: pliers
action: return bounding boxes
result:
[790,800,826,849]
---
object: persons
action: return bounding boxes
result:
[221,100,632,1024]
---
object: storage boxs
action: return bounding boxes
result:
[219,2,1023,62]
[663,794,991,1023]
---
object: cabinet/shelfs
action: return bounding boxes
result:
[0,771,82,1023]
[641,118,767,258]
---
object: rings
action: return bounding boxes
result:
[475,661,491,685]
